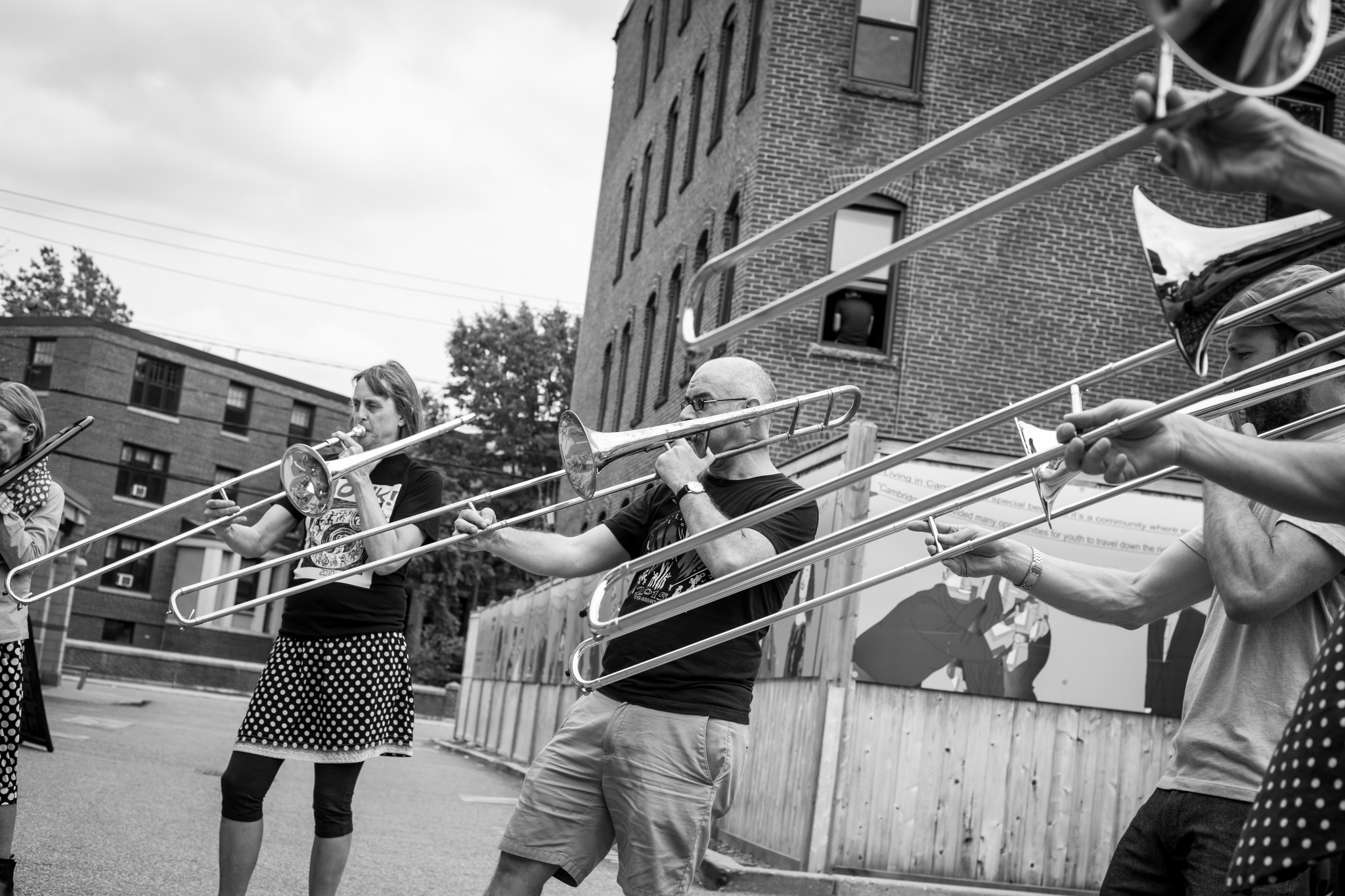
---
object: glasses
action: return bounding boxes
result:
[681,396,748,411]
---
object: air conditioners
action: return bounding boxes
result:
[116,573,134,587]
[130,484,147,498]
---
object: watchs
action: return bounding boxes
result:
[673,481,705,506]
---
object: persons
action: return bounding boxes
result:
[204,360,443,896]
[906,265,1345,896]
[452,357,820,896]
[0,381,65,896]
[1055,72,1345,896]
[833,290,874,346]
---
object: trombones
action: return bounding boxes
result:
[5,412,477,606]
[586,186,1345,638]
[0,415,95,493]
[680,2,1345,352]
[170,384,861,626]
[568,359,1344,694]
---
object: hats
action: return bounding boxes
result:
[1218,264,1345,353]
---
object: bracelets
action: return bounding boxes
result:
[1012,546,1043,592]
